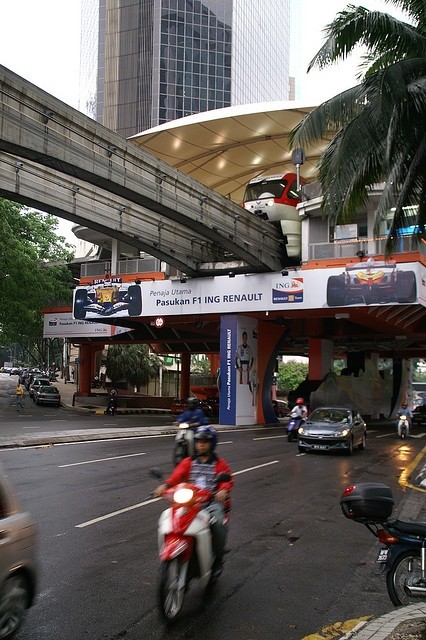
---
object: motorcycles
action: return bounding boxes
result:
[396,413,411,440]
[106,390,116,416]
[339,481,425,608]
[143,465,232,623]
[166,420,202,465]
[285,413,303,442]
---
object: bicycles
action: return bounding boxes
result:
[90,380,101,389]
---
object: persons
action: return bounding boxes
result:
[287,397,308,432]
[14,381,24,411]
[151,428,236,603]
[109,388,118,417]
[173,396,209,464]
[397,399,413,436]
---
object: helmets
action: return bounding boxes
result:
[187,395,199,405]
[194,425,217,450]
[401,401,408,406]
[296,397,304,404]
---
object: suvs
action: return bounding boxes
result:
[24,373,42,391]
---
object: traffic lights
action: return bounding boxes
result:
[290,148,305,165]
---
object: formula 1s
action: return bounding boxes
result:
[326,257,416,306]
[72,268,142,319]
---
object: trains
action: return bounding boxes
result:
[240,172,308,223]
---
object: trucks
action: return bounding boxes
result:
[3,362,12,372]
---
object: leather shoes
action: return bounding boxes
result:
[211,558,223,579]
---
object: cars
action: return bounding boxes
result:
[205,397,218,415]
[34,385,60,408]
[296,405,367,456]
[170,397,212,414]
[17,371,32,384]
[9,368,18,376]
[28,377,52,398]
[0,474,38,640]
[409,404,426,427]
